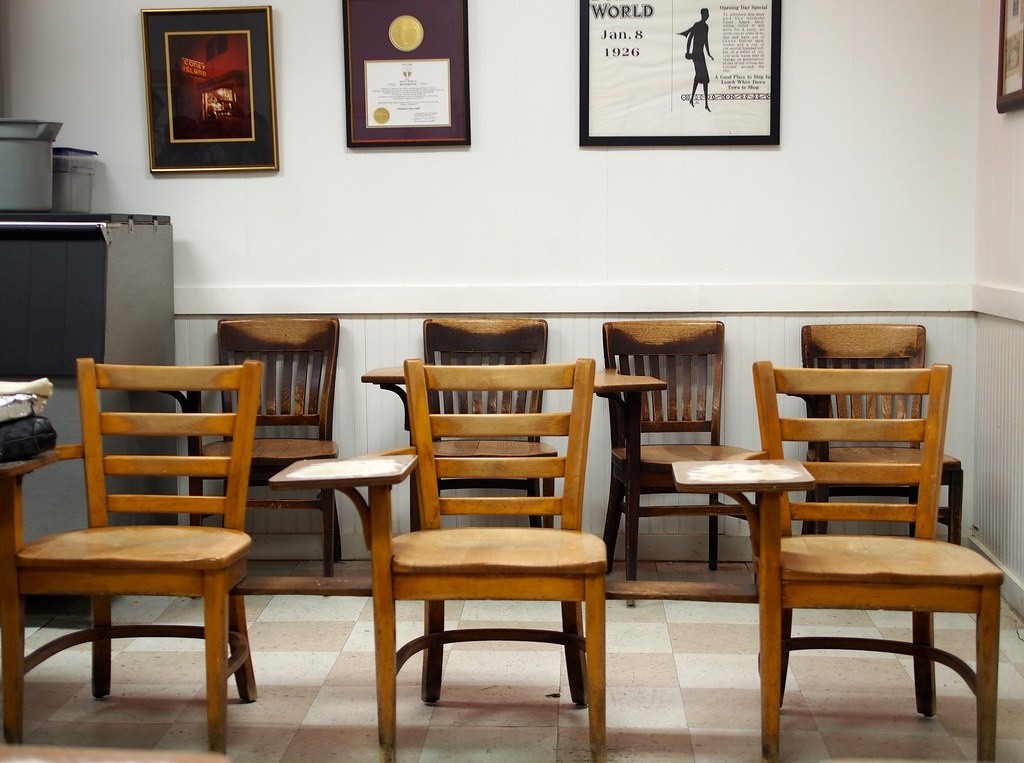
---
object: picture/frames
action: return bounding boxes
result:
[140,6,278,172]
[343,0,471,146]
[995,0,1023,113]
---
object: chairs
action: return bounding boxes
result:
[673,365,1006,762]
[799,322,966,547]
[0,358,266,752]
[593,321,766,583]
[270,357,610,763]
[360,318,559,532]
[159,314,339,576]
